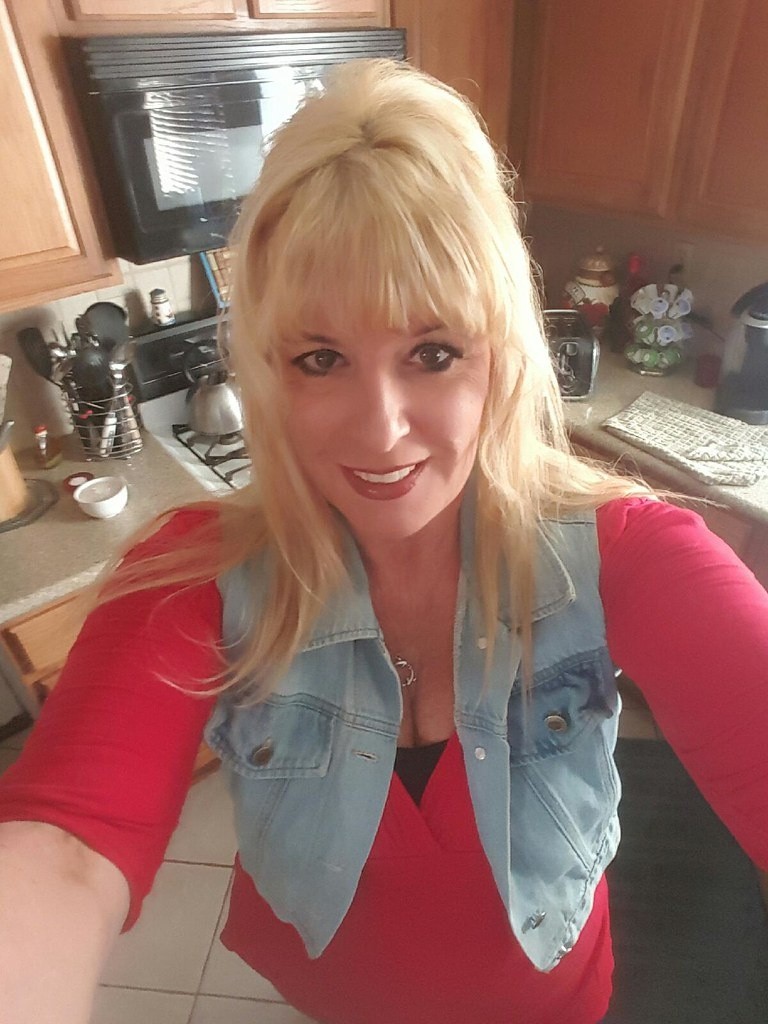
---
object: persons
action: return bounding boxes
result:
[0,56,768,1024]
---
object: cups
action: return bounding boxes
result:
[691,354,721,388]
[0,439,30,523]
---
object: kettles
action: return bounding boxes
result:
[180,338,245,437]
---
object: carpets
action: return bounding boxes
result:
[595,737,768,1024]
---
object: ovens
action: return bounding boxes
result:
[61,23,408,266]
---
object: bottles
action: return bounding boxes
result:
[149,288,177,327]
[609,253,649,353]
[31,424,63,470]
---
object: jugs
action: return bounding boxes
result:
[714,281,768,425]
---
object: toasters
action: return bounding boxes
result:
[540,309,601,402]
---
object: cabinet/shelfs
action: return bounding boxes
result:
[0,584,223,792]
[1,0,127,323]
[393,0,518,163]
[518,0,768,249]
[48,0,392,42]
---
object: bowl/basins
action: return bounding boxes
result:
[72,476,129,519]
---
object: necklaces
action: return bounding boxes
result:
[386,648,418,690]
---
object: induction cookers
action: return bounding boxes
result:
[122,307,258,499]
